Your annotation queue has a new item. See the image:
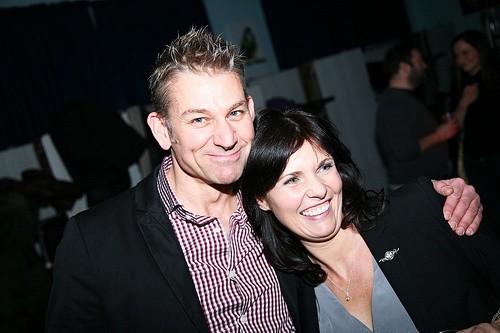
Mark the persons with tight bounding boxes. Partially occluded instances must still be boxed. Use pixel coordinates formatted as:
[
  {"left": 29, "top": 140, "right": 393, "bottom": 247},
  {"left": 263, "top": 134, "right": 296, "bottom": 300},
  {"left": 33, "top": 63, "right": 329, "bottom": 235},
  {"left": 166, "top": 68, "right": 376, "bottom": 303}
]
[{"left": 0, "top": 25, "right": 500, "bottom": 333}]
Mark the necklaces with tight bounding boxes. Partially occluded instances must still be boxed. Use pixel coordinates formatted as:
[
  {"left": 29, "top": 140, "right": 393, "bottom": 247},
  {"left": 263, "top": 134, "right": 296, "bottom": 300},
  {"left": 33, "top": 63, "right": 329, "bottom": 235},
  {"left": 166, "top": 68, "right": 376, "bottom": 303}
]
[{"left": 307, "top": 223, "right": 356, "bottom": 301}]
